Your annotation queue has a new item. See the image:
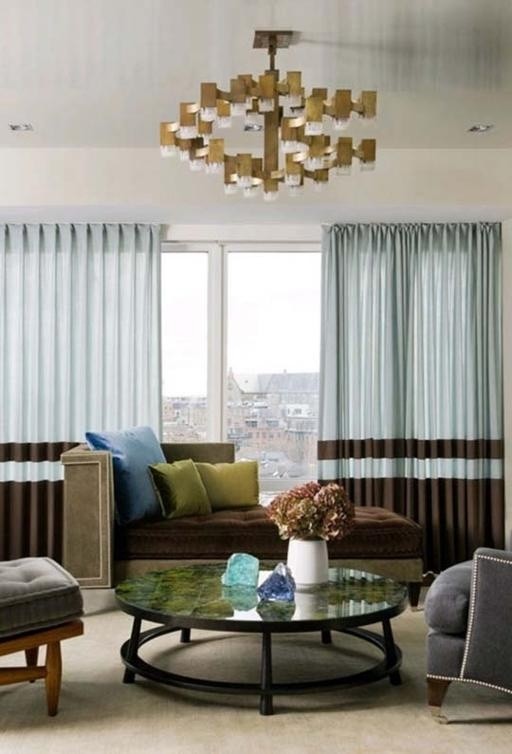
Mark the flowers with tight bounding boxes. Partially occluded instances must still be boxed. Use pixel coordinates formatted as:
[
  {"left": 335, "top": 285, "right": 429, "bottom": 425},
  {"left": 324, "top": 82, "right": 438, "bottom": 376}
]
[{"left": 265, "top": 477, "right": 359, "bottom": 543}]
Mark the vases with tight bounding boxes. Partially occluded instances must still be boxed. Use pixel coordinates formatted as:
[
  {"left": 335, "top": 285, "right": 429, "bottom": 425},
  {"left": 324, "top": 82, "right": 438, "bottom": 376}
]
[{"left": 285, "top": 536, "right": 329, "bottom": 587}]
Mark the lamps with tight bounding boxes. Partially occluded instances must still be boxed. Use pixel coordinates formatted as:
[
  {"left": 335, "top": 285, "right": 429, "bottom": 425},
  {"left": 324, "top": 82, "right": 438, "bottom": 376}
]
[{"left": 155, "top": 31, "right": 380, "bottom": 199}]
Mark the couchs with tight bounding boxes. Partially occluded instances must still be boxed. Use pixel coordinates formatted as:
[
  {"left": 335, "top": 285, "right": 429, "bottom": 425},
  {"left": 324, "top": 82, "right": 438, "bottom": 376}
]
[{"left": 60, "top": 435, "right": 426, "bottom": 616}]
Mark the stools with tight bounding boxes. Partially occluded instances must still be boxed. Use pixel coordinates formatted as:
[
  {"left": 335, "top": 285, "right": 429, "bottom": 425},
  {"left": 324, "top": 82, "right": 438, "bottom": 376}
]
[{"left": 0, "top": 556, "right": 85, "bottom": 716}]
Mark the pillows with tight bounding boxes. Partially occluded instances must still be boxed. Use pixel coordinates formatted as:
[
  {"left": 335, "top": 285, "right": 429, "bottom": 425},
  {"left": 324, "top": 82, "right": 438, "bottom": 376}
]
[{"left": 86, "top": 424, "right": 259, "bottom": 525}]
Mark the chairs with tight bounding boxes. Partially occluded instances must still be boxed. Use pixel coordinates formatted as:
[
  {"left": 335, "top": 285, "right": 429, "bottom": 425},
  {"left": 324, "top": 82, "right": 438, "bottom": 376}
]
[{"left": 423, "top": 540, "right": 511, "bottom": 718}]
